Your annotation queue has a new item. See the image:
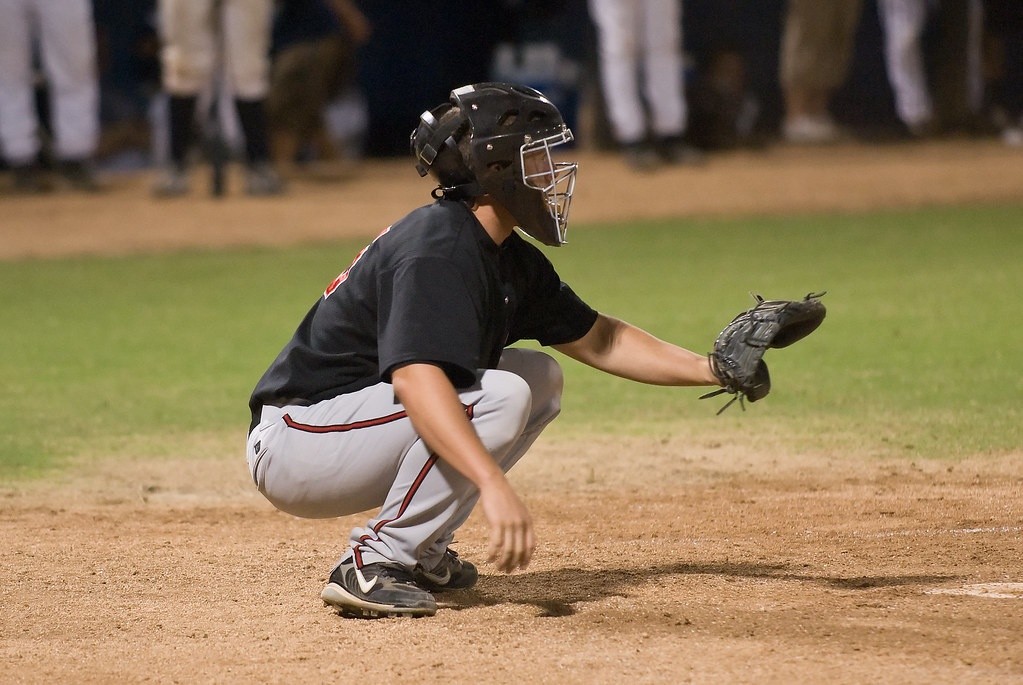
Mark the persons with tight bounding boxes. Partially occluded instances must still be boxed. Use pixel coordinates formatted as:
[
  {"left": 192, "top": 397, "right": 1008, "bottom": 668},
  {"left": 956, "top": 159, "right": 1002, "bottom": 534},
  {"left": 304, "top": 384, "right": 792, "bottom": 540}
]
[
  {"left": 2, "top": 1, "right": 1008, "bottom": 202},
  {"left": 247, "top": 75, "right": 788, "bottom": 622}
]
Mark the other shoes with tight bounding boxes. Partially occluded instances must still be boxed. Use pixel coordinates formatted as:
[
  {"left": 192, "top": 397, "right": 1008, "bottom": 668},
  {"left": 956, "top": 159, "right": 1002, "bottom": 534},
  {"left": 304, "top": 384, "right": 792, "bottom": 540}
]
[
  {"left": 623, "top": 138, "right": 703, "bottom": 175},
  {"left": 12, "top": 159, "right": 108, "bottom": 196},
  {"left": 783, "top": 115, "right": 840, "bottom": 143}
]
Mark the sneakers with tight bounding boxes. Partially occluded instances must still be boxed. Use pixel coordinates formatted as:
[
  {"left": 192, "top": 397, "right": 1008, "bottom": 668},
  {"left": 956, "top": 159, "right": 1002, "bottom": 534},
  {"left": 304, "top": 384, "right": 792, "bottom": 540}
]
[
  {"left": 321, "top": 548, "right": 436, "bottom": 616},
  {"left": 415, "top": 541, "right": 478, "bottom": 591}
]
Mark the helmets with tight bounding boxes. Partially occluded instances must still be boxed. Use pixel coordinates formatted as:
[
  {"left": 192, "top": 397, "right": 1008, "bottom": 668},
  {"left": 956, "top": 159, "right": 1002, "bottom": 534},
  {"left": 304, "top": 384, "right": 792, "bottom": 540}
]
[{"left": 410, "top": 81, "right": 578, "bottom": 247}]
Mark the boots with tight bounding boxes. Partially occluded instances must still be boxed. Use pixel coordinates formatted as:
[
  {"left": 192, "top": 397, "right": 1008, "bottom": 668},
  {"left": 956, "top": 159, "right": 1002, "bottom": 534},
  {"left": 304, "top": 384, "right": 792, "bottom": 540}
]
[
  {"left": 233, "top": 94, "right": 288, "bottom": 195},
  {"left": 157, "top": 93, "right": 196, "bottom": 199}
]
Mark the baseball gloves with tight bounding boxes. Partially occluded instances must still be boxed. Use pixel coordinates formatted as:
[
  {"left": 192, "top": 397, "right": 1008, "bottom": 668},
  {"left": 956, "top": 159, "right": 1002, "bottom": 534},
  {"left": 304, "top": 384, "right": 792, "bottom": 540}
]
[{"left": 698, "top": 288, "right": 833, "bottom": 420}]
